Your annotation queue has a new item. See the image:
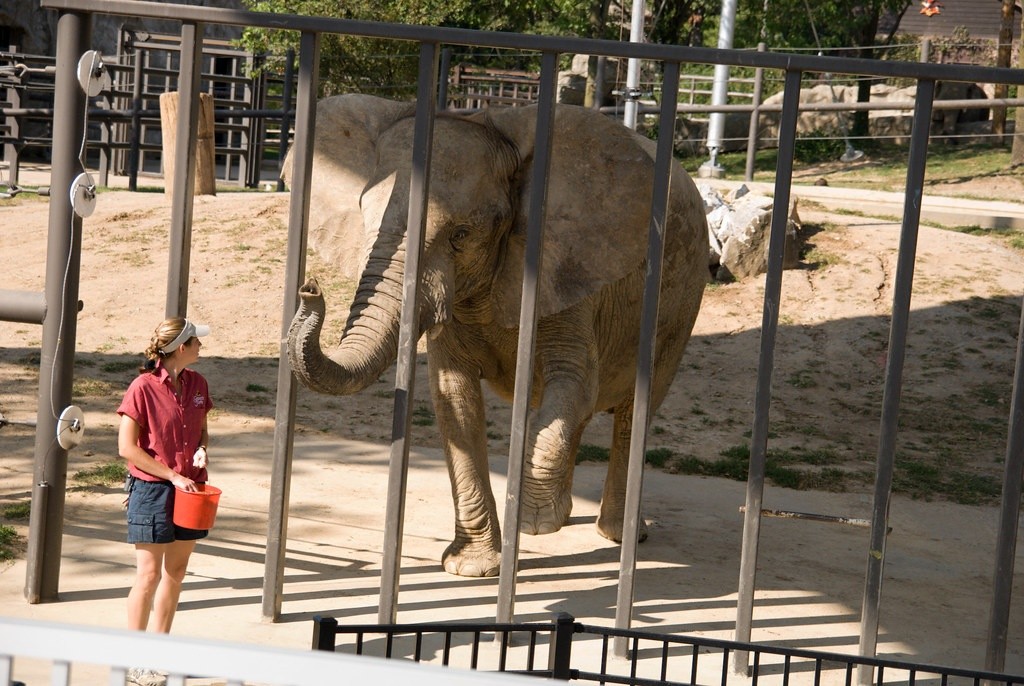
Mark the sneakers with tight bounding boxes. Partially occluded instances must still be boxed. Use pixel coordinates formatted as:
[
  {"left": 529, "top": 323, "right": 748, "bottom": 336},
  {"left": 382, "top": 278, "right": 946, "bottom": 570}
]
[{"left": 126, "top": 664, "right": 170, "bottom": 686}]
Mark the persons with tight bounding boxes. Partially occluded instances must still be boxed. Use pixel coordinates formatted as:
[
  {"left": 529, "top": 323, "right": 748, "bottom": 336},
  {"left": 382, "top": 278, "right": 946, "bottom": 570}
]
[{"left": 116, "top": 317, "right": 216, "bottom": 686}]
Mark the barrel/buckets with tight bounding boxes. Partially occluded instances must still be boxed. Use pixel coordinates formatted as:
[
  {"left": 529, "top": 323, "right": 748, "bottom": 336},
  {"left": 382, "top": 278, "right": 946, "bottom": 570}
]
[{"left": 172, "top": 464, "right": 222, "bottom": 530}]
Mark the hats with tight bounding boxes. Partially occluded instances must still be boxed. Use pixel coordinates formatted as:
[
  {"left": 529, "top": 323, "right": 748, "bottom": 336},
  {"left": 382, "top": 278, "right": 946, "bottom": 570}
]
[{"left": 158, "top": 318, "right": 210, "bottom": 353}]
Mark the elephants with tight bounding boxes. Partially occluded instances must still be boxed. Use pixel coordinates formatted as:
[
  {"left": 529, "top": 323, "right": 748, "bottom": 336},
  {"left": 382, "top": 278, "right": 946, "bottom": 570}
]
[{"left": 279, "top": 90, "right": 711, "bottom": 577}]
